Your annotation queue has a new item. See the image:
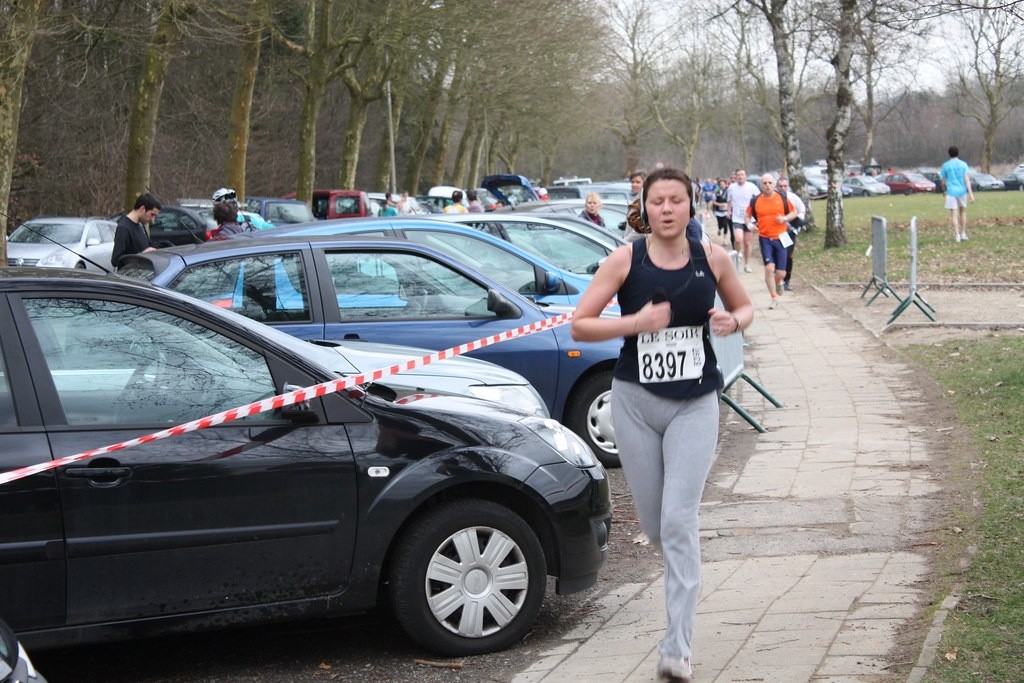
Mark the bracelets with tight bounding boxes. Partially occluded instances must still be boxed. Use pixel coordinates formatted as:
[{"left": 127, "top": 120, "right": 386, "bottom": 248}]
[
  {"left": 633, "top": 312, "right": 639, "bottom": 336},
  {"left": 733, "top": 315, "right": 740, "bottom": 332},
  {"left": 784, "top": 217, "right": 787, "bottom": 222},
  {"left": 747, "top": 223, "right": 754, "bottom": 230}
]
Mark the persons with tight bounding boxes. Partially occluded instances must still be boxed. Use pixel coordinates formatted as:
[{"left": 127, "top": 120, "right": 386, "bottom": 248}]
[
  {"left": 538, "top": 187, "right": 551, "bottom": 201},
  {"left": 941, "top": 146, "right": 975, "bottom": 242},
  {"left": 571, "top": 169, "right": 754, "bottom": 683},
  {"left": 848, "top": 166, "right": 895, "bottom": 177},
  {"left": 775, "top": 178, "right": 807, "bottom": 291},
  {"left": 692, "top": 173, "right": 743, "bottom": 259},
  {"left": 578, "top": 192, "right": 606, "bottom": 229},
  {"left": 111, "top": 193, "right": 162, "bottom": 271},
  {"left": 727, "top": 169, "right": 761, "bottom": 274},
  {"left": 464, "top": 190, "right": 483, "bottom": 213},
  {"left": 496, "top": 200, "right": 506, "bottom": 209},
  {"left": 744, "top": 174, "right": 798, "bottom": 309},
  {"left": 378, "top": 190, "right": 424, "bottom": 217},
  {"left": 624, "top": 170, "right": 651, "bottom": 243},
  {"left": 209, "top": 198, "right": 248, "bottom": 237},
  {"left": 443, "top": 191, "right": 469, "bottom": 214}
]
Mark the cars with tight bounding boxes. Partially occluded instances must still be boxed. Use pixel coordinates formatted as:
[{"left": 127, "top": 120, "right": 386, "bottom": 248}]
[
  {"left": 1, "top": 173, "right": 648, "bottom": 472},
  {"left": 744, "top": 156, "right": 1024, "bottom": 204},
  {"left": 1, "top": 267, "right": 617, "bottom": 667}
]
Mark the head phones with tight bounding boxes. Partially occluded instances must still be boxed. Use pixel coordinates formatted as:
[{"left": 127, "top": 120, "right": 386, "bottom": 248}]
[{"left": 642, "top": 182, "right": 696, "bottom": 224}]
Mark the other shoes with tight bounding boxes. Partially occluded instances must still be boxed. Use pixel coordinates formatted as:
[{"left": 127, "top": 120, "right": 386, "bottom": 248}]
[
  {"left": 776, "top": 280, "right": 784, "bottom": 296},
  {"left": 659, "top": 655, "right": 692, "bottom": 682},
  {"left": 784, "top": 281, "right": 793, "bottom": 291},
  {"left": 744, "top": 265, "right": 752, "bottom": 273},
  {"left": 769, "top": 299, "right": 778, "bottom": 309},
  {"left": 962, "top": 234, "right": 969, "bottom": 241},
  {"left": 955, "top": 236, "right": 962, "bottom": 242}
]
